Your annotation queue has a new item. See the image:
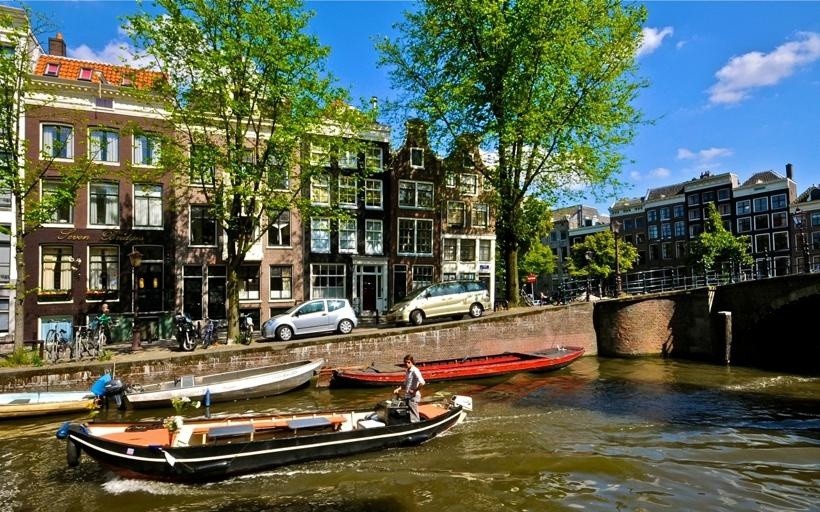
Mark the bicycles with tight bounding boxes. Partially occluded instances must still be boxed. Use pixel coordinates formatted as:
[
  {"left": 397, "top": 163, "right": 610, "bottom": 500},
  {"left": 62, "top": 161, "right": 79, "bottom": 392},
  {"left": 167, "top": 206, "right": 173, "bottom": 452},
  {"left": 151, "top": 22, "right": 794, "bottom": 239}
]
[
  {"left": 46, "top": 317, "right": 110, "bottom": 365},
  {"left": 201, "top": 317, "right": 220, "bottom": 349},
  {"left": 239, "top": 311, "right": 256, "bottom": 346}
]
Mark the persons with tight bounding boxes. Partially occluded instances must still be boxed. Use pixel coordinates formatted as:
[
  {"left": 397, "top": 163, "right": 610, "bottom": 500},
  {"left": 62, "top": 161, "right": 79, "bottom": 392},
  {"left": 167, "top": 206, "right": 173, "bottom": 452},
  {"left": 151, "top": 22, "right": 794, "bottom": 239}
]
[{"left": 394, "top": 354, "right": 425, "bottom": 422}]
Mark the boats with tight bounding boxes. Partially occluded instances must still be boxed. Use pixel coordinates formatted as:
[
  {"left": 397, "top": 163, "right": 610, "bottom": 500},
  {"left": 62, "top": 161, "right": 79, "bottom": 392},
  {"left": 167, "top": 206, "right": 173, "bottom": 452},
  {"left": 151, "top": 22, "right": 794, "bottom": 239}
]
[
  {"left": 0, "top": 390, "right": 104, "bottom": 420},
  {"left": 54, "top": 393, "right": 475, "bottom": 485},
  {"left": 332, "top": 345, "right": 586, "bottom": 389},
  {"left": 103, "top": 356, "right": 330, "bottom": 411}
]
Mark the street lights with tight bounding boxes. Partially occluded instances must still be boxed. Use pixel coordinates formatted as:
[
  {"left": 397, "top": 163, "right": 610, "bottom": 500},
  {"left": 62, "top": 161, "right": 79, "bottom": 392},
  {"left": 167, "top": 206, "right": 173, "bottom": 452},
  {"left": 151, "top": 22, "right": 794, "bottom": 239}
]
[
  {"left": 611, "top": 219, "right": 623, "bottom": 299},
  {"left": 791, "top": 206, "right": 814, "bottom": 273},
  {"left": 127, "top": 246, "right": 144, "bottom": 352}
]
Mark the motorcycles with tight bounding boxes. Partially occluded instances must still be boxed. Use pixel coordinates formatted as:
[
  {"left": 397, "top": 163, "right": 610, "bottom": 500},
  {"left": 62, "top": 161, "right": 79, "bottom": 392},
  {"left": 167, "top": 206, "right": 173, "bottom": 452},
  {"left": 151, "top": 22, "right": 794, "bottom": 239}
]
[{"left": 173, "top": 312, "right": 200, "bottom": 352}]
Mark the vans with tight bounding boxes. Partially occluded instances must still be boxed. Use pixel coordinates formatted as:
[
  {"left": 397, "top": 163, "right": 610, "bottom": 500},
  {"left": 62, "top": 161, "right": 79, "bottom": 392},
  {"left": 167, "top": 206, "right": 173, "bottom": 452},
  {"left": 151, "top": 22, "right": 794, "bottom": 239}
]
[{"left": 384, "top": 279, "right": 491, "bottom": 327}]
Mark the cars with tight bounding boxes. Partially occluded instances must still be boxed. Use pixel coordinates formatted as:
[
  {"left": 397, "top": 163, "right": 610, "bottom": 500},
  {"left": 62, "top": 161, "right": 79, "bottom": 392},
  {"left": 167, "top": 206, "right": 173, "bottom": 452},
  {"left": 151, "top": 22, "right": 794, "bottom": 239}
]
[{"left": 260, "top": 296, "right": 360, "bottom": 342}]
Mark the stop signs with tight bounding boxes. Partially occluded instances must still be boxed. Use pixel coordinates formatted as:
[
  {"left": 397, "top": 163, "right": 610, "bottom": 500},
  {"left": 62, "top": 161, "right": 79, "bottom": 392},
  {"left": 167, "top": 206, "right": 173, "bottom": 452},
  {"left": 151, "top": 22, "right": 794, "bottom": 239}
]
[{"left": 527, "top": 274, "right": 536, "bottom": 283}]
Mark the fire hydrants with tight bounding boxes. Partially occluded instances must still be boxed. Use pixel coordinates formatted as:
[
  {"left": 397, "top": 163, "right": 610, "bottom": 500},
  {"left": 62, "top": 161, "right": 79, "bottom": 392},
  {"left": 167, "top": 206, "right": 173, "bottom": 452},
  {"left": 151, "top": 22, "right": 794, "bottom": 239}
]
[{"left": 130, "top": 320, "right": 145, "bottom": 352}]
